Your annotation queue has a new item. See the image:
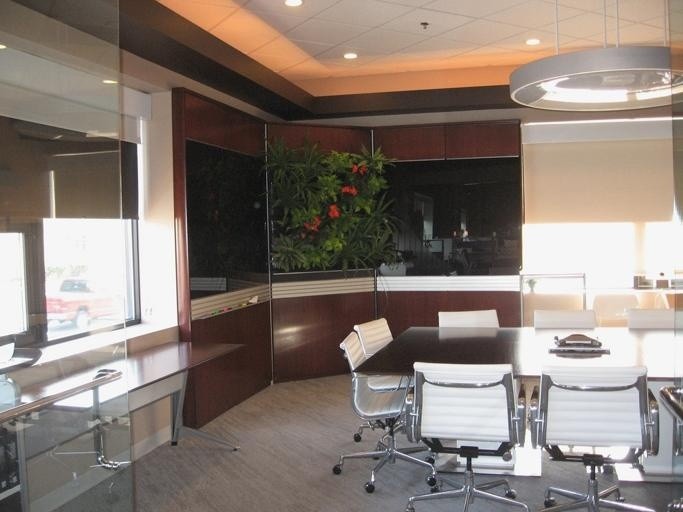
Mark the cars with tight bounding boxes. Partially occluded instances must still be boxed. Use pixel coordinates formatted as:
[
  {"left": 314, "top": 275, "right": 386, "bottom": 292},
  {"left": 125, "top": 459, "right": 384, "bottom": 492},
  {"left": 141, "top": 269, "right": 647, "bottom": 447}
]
[{"left": 45, "top": 276, "right": 116, "bottom": 330}]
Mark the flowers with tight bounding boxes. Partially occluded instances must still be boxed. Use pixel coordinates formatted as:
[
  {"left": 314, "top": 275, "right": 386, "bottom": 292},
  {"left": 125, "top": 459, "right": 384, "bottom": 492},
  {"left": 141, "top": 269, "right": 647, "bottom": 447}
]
[{"left": 256, "top": 134, "right": 400, "bottom": 272}]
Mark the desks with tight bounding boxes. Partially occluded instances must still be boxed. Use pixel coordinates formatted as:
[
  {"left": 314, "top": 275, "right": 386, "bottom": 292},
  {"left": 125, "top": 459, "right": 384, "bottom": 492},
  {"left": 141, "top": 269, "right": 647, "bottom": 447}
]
[
  {"left": 354, "top": 326, "right": 682, "bottom": 484},
  {"left": 19, "top": 340, "right": 245, "bottom": 470}
]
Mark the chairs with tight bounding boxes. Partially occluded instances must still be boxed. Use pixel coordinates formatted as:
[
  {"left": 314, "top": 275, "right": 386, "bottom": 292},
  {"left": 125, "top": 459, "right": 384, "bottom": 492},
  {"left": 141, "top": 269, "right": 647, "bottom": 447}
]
[
  {"left": 535, "top": 309, "right": 597, "bottom": 327},
  {"left": 354, "top": 319, "right": 416, "bottom": 443},
  {"left": 379, "top": 225, "right": 522, "bottom": 275},
  {"left": 660, "top": 386, "right": 682, "bottom": 509},
  {"left": 627, "top": 310, "right": 677, "bottom": 327},
  {"left": 190, "top": 250, "right": 227, "bottom": 292},
  {"left": 405, "top": 361, "right": 530, "bottom": 512},
  {"left": 439, "top": 310, "right": 498, "bottom": 328},
  {"left": 339, "top": 331, "right": 436, "bottom": 492},
  {"left": 537, "top": 366, "right": 657, "bottom": 511}
]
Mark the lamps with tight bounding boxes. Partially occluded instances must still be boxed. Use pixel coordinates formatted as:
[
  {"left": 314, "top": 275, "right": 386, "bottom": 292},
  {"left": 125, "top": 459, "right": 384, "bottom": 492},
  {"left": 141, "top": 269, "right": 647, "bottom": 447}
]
[{"left": 510, "top": 0, "right": 683, "bottom": 112}]
